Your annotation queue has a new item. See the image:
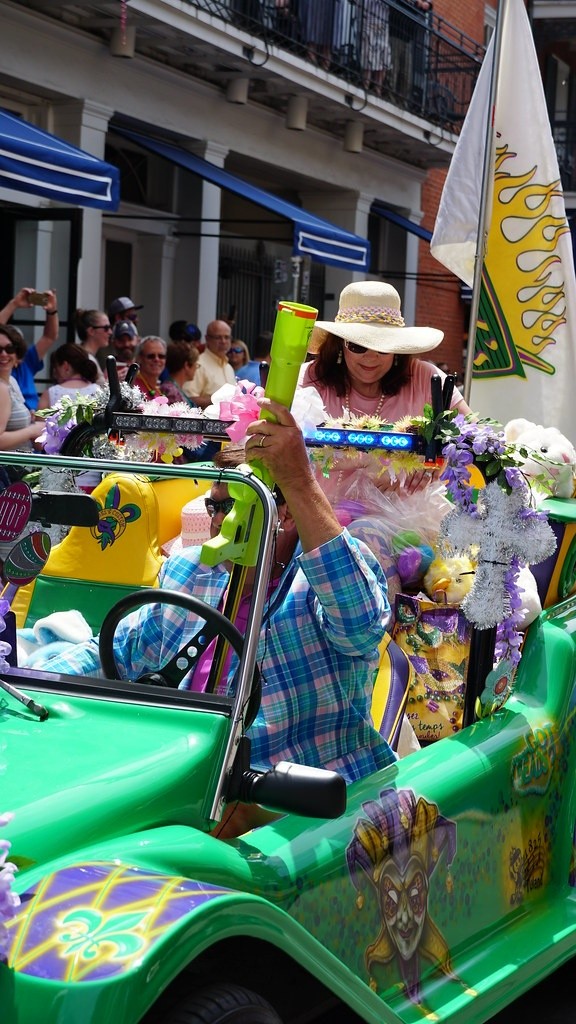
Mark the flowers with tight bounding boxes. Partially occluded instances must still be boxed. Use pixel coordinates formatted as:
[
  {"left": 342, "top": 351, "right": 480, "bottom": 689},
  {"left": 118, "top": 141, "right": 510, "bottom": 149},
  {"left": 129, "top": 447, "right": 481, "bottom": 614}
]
[
  {"left": 36, "top": 409, "right": 70, "bottom": 453},
  {"left": 444, "top": 414, "right": 551, "bottom": 666},
  {"left": 319, "top": 415, "right": 429, "bottom": 475}
]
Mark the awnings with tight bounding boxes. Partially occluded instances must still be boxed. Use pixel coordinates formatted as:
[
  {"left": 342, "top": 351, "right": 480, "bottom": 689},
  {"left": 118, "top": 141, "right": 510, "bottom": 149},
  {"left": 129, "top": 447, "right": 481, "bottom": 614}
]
[
  {"left": 370, "top": 205, "right": 465, "bottom": 284},
  {"left": 0, "top": 108, "right": 120, "bottom": 213},
  {"left": 108, "top": 128, "right": 371, "bottom": 274}
]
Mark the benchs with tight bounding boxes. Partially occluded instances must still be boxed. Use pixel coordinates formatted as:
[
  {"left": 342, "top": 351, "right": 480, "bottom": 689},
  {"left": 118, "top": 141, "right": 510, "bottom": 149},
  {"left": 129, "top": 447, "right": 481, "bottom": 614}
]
[{"left": 21, "top": 577, "right": 405, "bottom": 764}]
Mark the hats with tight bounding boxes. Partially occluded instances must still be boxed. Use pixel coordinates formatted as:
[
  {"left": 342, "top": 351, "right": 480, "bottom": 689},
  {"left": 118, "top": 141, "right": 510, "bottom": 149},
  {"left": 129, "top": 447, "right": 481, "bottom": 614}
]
[
  {"left": 108, "top": 297, "right": 144, "bottom": 316},
  {"left": 161, "top": 494, "right": 213, "bottom": 557},
  {"left": 307, "top": 281, "right": 445, "bottom": 355},
  {"left": 112, "top": 320, "right": 138, "bottom": 339}
]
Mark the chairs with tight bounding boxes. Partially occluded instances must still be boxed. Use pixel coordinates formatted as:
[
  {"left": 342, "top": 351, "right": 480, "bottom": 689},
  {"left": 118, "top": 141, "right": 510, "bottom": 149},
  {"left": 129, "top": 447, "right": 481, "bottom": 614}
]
[{"left": 280, "top": 14, "right": 449, "bottom": 125}]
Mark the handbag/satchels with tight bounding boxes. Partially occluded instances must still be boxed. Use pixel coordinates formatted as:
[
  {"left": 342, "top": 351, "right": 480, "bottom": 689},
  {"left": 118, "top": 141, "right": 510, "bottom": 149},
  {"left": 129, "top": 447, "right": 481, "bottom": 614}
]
[{"left": 392, "top": 592, "right": 497, "bottom": 743}]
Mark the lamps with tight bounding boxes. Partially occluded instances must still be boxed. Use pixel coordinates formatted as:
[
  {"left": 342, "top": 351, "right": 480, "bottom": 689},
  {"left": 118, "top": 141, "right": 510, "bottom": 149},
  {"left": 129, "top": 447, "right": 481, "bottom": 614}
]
[
  {"left": 283, "top": 92, "right": 307, "bottom": 134},
  {"left": 226, "top": 78, "right": 249, "bottom": 104},
  {"left": 108, "top": 26, "right": 135, "bottom": 58},
  {"left": 344, "top": 121, "right": 367, "bottom": 151}
]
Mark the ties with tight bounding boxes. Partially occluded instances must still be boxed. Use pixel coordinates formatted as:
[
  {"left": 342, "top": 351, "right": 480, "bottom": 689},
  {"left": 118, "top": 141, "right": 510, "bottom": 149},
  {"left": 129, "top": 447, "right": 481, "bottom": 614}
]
[{"left": 186, "top": 577, "right": 281, "bottom": 695}]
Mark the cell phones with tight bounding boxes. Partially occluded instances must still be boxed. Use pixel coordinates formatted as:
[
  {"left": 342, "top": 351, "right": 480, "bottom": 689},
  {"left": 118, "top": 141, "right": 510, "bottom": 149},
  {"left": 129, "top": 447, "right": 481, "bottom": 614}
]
[
  {"left": 227, "top": 305, "right": 236, "bottom": 320},
  {"left": 27, "top": 291, "right": 49, "bottom": 306}
]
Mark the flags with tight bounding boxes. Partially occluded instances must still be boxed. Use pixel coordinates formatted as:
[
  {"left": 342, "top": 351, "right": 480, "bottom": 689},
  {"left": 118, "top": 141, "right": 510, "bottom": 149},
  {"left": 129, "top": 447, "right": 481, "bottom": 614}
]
[{"left": 429, "top": 0, "right": 576, "bottom": 450}]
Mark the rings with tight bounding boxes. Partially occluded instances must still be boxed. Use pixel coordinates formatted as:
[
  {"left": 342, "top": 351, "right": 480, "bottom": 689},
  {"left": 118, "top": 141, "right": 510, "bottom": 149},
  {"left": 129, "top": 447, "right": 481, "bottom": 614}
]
[
  {"left": 425, "top": 471, "right": 432, "bottom": 478},
  {"left": 260, "top": 435, "right": 266, "bottom": 446}
]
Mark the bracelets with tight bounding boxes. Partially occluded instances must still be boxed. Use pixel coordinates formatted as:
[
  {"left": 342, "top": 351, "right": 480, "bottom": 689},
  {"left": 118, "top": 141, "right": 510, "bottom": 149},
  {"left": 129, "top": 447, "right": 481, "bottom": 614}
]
[{"left": 46, "top": 311, "right": 58, "bottom": 314}]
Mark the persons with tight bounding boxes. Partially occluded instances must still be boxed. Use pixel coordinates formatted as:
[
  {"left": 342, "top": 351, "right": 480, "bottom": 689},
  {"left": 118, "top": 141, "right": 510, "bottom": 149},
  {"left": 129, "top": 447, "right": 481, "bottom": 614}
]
[
  {"left": 34, "top": 398, "right": 392, "bottom": 840},
  {"left": 38, "top": 343, "right": 104, "bottom": 410},
  {"left": 357, "top": 0, "right": 429, "bottom": 95},
  {"left": 0, "top": 324, "right": 46, "bottom": 452},
  {"left": 0, "top": 288, "right": 59, "bottom": 411},
  {"left": 104, "top": 297, "right": 202, "bottom": 408},
  {"left": 299, "top": 0, "right": 336, "bottom": 72},
  {"left": 185, "top": 320, "right": 237, "bottom": 405},
  {"left": 228, "top": 339, "right": 260, "bottom": 386},
  {"left": 291, "top": 280, "right": 479, "bottom": 606},
  {"left": 73, "top": 309, "right": 112, "bottom": 383}
]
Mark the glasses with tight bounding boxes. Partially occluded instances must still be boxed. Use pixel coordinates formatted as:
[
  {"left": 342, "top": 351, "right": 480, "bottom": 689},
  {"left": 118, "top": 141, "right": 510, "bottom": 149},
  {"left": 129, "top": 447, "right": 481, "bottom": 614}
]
[
  {"left": 208, "top": 335, "right": 232, "bottom": 341},
  {"left": 228, "top": 348, "right": 244, "bottom": 353},
  {"left": 0, "top": 344, "right": 16, "bottom": 354},
  {"left": 192, "top": 362, "right": 201, "bottom": 367},
  {"left": 120, "top": 314, "right": 136, "bottom": 320},
  {"left": 92, "top": 325, "right": 111, "bottom": 330},
  {"left": 142, "top": 353, "right": 166, "bottom": 359},
  {"left": 344, "top": 340, "right": 388, "bottom": 354},
  {"left": 205, "top": 498, "right": 236, "bottom": 517}
]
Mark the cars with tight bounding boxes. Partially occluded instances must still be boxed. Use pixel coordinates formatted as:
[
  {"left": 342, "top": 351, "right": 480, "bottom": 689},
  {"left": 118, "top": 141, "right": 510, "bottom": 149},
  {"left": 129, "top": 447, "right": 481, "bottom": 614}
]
[{"left": 1, "top": 453, "right": 576, "bottom": 1024}]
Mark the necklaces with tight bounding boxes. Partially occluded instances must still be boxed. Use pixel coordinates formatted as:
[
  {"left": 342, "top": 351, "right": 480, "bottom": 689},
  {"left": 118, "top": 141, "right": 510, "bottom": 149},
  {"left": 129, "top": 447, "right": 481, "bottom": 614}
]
[
  {"left": 335, "top": 392, "right": 385, "bottom": 501},
  {"left": 138, "top": 374, "right": 157, "bottom": 395}
]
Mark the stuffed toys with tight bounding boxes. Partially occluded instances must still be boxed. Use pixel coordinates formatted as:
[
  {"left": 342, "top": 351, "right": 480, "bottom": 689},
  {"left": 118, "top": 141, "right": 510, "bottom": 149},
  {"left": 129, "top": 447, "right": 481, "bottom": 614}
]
[
  {"left": 426, "top": 537, "right": 542, "bottom": 628},
  {"left": 505, "top": 418, "right": 576, "bottom": 499}
]
